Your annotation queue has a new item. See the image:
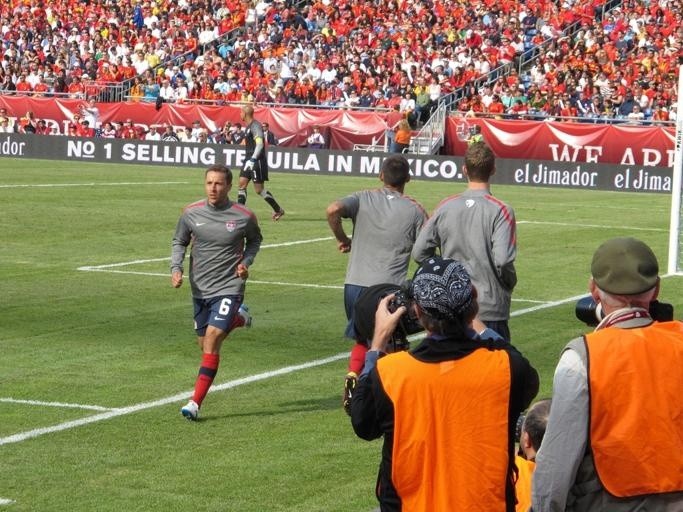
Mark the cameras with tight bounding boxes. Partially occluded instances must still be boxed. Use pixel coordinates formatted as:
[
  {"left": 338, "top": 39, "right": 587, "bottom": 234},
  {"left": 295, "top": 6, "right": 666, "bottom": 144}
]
[
  {"left": 378, "top": 281, "right": 424, "bottom": 336},
  {"left": 574, "top": 295, "right": 675, "bottom": 325}
]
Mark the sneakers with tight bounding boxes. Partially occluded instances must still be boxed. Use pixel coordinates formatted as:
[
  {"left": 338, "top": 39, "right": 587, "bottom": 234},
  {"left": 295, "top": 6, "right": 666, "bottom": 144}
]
[
  {"left": 238, "top": 304, "right": 251, "bottom": 327},
  {"left": 182, "top": 400, "right": 199, "bottom": 420},
  {"left": 272, "top": 208, "right": 284, "bottom": 220},
  {"left": 344, "top": 371, "right": 357, "bottom": 415}
]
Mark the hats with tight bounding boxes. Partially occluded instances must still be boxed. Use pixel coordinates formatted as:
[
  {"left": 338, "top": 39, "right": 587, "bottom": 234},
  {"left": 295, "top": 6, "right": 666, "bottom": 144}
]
[
  {"left": 411, "top": 256, "right": 472, "bottom": 319},
  {"left": 591, "top": 238, "right": 658, "bottom": 295}
]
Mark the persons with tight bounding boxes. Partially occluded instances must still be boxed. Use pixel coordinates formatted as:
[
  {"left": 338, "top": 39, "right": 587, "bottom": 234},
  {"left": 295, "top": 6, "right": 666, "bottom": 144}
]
[
  {"left": 171, "top": 166, "right": 263, "bottom": 420},
  {"left": 234, "top": 107, "right": 286, "bottom": 220},
  {"left": 326, "top": 155, "right": 429, "bottom": 415},
  {"left": 531, "top": 237, "right": 682, "bottom": 510},
  {"left": 411, "top": 142, "right": 517, "bottom": 345},
  {"left": 350, "top": 258, "right": 539, "bottom": 509},
  {"left": 0, "top": 0, "right": 682, "bottom": 153},
  {"left": 512, "top": 401, "right": 553, "bottom": 509}
]
